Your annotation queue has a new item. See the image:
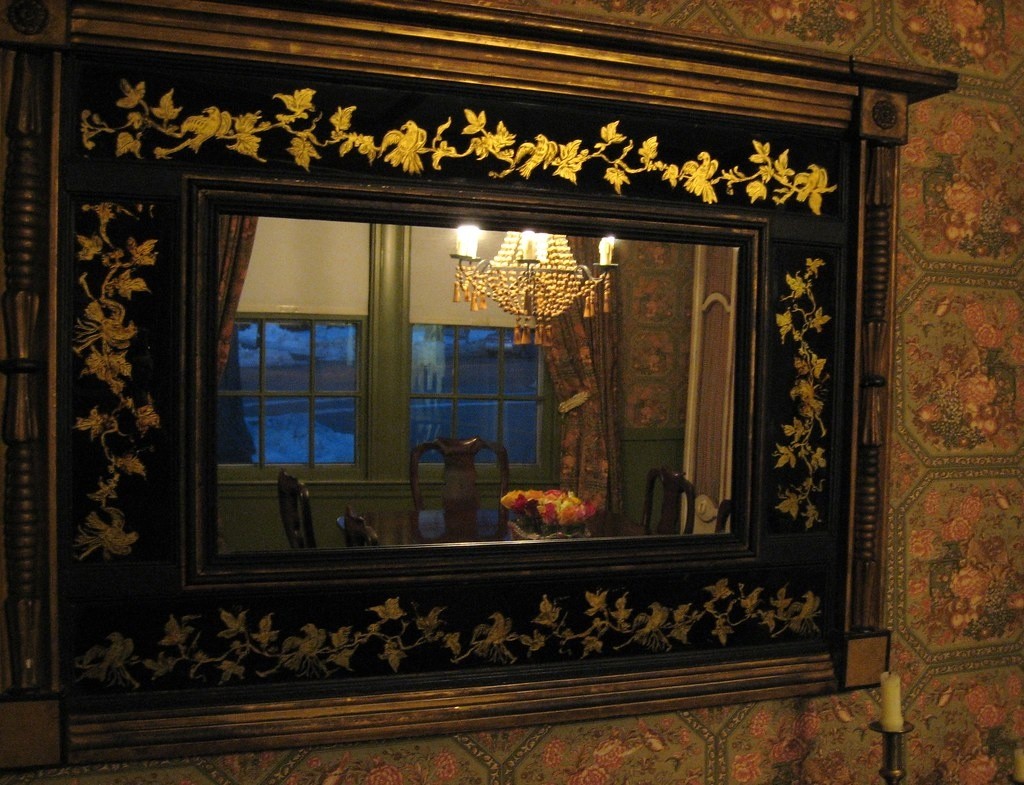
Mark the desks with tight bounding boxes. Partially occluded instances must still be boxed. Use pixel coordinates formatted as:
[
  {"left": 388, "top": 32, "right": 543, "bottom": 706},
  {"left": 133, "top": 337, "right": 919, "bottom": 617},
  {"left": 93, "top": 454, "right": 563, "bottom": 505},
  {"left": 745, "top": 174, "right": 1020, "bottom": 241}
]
[{"left": 334, "top": 513, "right": 645, "bottom": 542}]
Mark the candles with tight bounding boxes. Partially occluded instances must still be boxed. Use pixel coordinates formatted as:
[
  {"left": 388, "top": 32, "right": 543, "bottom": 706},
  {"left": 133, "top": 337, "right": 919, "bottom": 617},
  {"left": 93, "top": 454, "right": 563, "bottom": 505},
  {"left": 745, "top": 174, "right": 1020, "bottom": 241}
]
[{"left": 881, "top": 670, "right": 905, "bottom": 728}]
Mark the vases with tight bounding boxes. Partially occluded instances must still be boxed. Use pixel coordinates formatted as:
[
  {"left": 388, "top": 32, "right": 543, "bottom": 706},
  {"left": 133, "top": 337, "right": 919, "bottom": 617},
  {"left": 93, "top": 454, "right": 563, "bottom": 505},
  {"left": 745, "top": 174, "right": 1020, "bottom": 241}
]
[{"left": 506, "top": 519, "right": 595, "bottom": 541}]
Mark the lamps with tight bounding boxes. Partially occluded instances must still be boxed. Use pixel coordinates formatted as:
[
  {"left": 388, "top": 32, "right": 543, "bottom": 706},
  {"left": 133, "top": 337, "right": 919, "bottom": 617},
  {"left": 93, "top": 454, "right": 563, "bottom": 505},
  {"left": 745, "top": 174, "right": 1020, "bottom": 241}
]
[{"left": 448, "top": 223, "right": 617, "bottom": 346}]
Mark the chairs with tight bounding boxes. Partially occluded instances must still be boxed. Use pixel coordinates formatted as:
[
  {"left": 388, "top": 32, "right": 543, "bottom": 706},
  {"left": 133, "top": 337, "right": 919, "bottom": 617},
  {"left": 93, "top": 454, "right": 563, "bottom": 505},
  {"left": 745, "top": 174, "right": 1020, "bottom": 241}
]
[
  {"left": 641, "top": 469, "right": 700, "bottom": 536},
  {"left": 409, "top": 437, "right": 510, "bottom": 512},
  {"left": 279, "top": 470, "right": 316, "bottom": 547},
  {"left": 345, "top": 504, "right": 381, "bottom": 546}
]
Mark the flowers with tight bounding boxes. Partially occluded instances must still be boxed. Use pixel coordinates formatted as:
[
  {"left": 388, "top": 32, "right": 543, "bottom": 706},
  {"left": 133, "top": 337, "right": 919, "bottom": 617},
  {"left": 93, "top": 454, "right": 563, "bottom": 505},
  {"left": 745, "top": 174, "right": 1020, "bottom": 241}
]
[{"left": 500, "top": 489, "right": 606, "bottom": 530}]
